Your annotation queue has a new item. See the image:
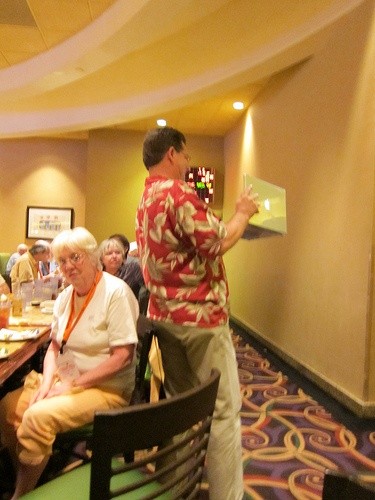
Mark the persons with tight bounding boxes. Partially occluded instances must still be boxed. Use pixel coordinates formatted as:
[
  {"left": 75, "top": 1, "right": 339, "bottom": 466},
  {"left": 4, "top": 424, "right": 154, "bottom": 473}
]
[
  {"left": 0, "top": 233, "right": 145, "bottom": 302},
  {"left": 0, "top": 227, "right": 140, "bottom": 500},
  {"left": 135, "top": 128, "right": 261, "bottom": 500}
]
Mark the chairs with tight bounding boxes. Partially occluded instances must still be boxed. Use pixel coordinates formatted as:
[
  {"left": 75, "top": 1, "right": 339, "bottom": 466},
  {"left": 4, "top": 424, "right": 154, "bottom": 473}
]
[
  {"left": 35, "top": 315, "right": 155, "bottom": 489},
  {"left": 16, "top": 368, "right": 221, "bottom": 500}
]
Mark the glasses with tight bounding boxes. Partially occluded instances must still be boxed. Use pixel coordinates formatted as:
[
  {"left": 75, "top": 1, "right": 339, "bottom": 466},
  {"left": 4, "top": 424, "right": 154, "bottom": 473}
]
[{"left": 55, "top": 253, "right": 87, "bottom": 267}]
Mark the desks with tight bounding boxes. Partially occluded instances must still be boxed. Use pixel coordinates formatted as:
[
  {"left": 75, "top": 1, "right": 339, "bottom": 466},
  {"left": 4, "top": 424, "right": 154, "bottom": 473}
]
[{"left": 0, "top": 324, "right": 52, "bottom": 483}]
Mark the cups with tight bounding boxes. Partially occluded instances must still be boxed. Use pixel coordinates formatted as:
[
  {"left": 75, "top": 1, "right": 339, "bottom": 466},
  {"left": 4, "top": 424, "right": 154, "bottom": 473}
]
[
  {"left": 0, "top": 303, "right": 12, "bottom": 330},
  {"left": 12, "top": 297, "right": 23, "bottom": 317},
  {"left": 21, "top": 277, "right": 59, "bottom": 312}
]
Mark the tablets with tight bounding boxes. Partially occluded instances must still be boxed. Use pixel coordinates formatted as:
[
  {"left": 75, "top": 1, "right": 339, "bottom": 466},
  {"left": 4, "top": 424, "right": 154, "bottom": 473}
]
[{"left": 243, "top": 174, "right": 287, "bottom": 235}]
